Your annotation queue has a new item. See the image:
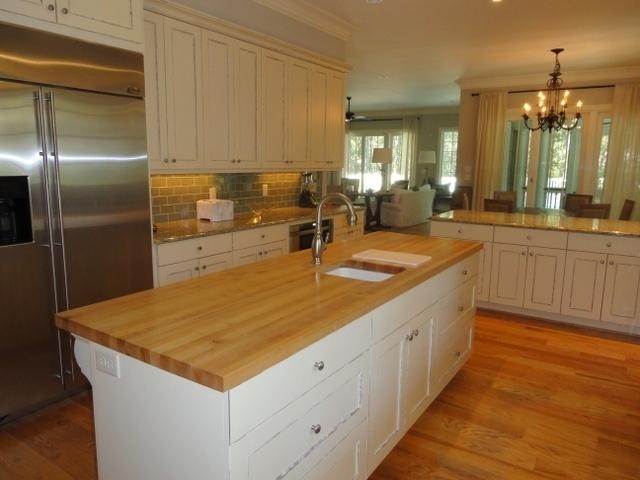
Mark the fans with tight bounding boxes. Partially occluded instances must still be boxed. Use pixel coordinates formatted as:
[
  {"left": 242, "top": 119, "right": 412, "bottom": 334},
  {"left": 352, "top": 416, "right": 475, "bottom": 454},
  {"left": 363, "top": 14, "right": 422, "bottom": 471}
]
[{"left": 343, "top": 93, "right": 370, "bottom": 123}]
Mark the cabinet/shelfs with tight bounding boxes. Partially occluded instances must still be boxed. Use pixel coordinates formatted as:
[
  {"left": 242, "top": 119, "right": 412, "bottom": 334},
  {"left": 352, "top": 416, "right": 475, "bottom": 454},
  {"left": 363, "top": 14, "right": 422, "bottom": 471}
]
[
  {"left": 366, "top": 277, "right": 432, "bottom": 478},
  {"left": 202, "top": 12, "right": 267, "bottom": 181},
  {"left": 230, "top": 224, "right": 294, "bottom": 268},
  {"left": 312, "top": 49, "right": 350, "bottom": 178},
  {"left": 331, "top": 212, "right": 365, "bottom": 242},
  {"left": 493, "top": 223, "right": 563, "bottom": 324},
  {"left": 75, "top": 317, "right": 366, "bottom": 479},
  {"left": 143, "top": 0, "right": 204, "bottom": 175},
  {"left": 148, "top": 228, "right": 231, "bottom": 287},
  {"left": 564, "top": 235, "right": 639, "bottom": 342},
  {"left": 427, "top": 253, "right": 483, "bottom": 405},
  {"left": 1, "top": 2, "right": 145, "bottom": 52},
  {"left": 265, "top": 33, "right": 313, "bottom": 178},
  {"left": 431, "top": 221, "right": 492, "bottom": 311}
]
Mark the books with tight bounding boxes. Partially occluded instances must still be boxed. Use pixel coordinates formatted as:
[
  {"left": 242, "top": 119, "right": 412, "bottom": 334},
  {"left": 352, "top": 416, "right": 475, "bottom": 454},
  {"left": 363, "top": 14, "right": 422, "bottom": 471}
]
[{"left": 351, "top": 248, "right": 432, "bottom": 266}]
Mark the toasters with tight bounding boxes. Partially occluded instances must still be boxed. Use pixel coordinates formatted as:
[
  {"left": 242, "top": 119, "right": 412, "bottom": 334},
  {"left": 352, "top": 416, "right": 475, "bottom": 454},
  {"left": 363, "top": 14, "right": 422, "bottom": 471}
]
[{"left": 196, "top": 199, "right": 234, "bottom": 222}]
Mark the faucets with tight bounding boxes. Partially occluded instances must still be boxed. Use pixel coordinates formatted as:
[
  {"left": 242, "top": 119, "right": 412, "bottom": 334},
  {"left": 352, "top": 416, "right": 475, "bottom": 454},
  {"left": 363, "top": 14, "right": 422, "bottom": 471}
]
[{"left": 311, "top": 192, "right": 357, "bottom": 266}]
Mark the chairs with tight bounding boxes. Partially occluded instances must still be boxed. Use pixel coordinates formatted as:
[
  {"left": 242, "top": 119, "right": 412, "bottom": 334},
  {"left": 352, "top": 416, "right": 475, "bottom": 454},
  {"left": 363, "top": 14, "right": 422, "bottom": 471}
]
[
  {"left": 482, "top": 188, "right": 636, "bottom": 222},
  {"left": 327, "top": 177, "right": 474, "bottom": 231}
]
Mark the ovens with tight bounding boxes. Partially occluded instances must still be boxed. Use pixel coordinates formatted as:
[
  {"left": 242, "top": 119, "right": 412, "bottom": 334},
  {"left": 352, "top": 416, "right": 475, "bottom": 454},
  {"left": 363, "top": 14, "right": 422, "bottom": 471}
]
[{"left": 289, "top": 218, "right": 334, "bottom": 253}]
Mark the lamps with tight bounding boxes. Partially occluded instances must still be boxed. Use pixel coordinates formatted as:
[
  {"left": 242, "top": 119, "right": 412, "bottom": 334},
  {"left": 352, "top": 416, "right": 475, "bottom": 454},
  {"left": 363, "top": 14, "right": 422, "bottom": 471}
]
[
  {"left": 371, "top": 147, "right": 393, "bottom": 190},
  {"left": 416, "top": 150, "right": 438, "bottom": 186},
  {"left": 520, "top": 47, "right": 586, "bottom": 135}
]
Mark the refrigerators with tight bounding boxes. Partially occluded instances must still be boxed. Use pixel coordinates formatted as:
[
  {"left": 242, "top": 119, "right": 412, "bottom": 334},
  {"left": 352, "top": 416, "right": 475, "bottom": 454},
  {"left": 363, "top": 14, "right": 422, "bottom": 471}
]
[{"left": 0, "top": 19, "right": 156, "bottom": 430}]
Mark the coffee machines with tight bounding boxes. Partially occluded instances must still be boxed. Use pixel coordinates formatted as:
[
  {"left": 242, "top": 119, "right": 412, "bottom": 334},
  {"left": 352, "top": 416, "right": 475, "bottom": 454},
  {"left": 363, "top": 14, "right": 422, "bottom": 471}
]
[{"left": 298, "top": 171, "right": 321, "bottom": 209}]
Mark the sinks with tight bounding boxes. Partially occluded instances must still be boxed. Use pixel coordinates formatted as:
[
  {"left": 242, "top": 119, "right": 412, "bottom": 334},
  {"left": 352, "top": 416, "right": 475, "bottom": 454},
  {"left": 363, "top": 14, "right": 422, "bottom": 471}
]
[{"left": 325, "top": 267, "right": 393, "bottom": 282}]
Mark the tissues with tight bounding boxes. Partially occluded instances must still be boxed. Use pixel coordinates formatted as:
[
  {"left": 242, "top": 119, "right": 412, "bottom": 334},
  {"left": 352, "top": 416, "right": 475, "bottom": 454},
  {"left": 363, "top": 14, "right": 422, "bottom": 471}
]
[{"left": 196, "top": 188, "right": 234, "bottom": 222}]
